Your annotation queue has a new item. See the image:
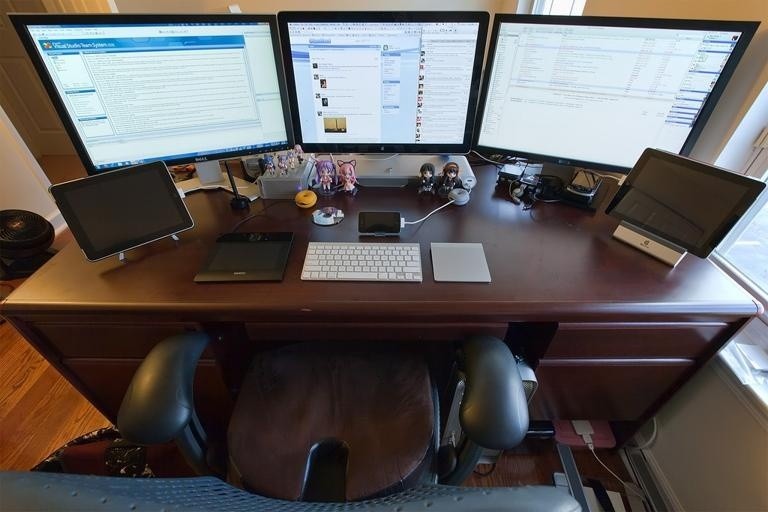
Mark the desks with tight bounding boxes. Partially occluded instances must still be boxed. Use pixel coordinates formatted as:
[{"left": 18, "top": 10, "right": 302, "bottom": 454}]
[{"left": 0, "top": 159, "right": 764, "bottom": 452}]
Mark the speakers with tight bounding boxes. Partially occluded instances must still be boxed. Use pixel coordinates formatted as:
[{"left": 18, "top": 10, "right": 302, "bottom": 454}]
[{"left": 439, "top": 354, "right": 539, "bottom": 479}]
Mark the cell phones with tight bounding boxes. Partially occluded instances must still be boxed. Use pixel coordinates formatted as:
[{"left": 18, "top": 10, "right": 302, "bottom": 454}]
[
  {"left": 567, "top": 171, "right": 602, "bottom": 197},
  {"left": 357, "top": 211, "right": 401, "bottom": 235}
]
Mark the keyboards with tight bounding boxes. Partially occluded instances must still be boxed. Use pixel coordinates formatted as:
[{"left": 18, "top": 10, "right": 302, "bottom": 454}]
[{"left": 300, "top": 242, "right": 423, "bottom": 283}]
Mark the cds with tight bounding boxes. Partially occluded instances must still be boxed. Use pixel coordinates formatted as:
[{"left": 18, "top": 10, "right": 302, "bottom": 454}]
[{"left": 311, "top": 206, "right": 344, "bottom": 226}]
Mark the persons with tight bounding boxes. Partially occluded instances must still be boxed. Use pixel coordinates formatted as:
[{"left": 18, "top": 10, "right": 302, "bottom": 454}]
[
  {"left": 416, "top": 163, "right": 436, "bottom": 195},
  {"left": 277, "top": 155, "right": 288, "bottom": 176},
  {"left": 294, "top": 145, "right": 304, "bottom": 164},
  {"left": 313, "top": 160, "right": 336, "bottom": 193},
  {"left": 287, "top": 149, "right": 296, "bottom": 169},
  {"left": 442, "top": 162, "right": 460, "bottom": 192},
  {"left": 337, "top": 159, "right": 360, "bottom": 195},
  {"left": 263, "top": 156, "right": 275, "bottom": 175}
]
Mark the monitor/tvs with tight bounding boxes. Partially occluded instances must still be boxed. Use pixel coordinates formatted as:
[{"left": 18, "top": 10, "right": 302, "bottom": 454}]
[
  {"left": 471, "top": 13, "right": 761, "bottom": 214},
  {"left": 604, "top": 148, "right": 767, "bottom": 268},
  {"left": 7, "top": 12, "right": 295, "bottom": 202},
  {"left": 276, "top": 10, "right": 489, "bottom": 188},
  {"left": 48, "top": 160, "right": 195, "bottom": 263}
]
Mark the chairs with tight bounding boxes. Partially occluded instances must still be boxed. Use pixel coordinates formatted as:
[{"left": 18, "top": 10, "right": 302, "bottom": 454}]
[{"left": 0, "top": 331, "right": 582, "bottom": 512}]
